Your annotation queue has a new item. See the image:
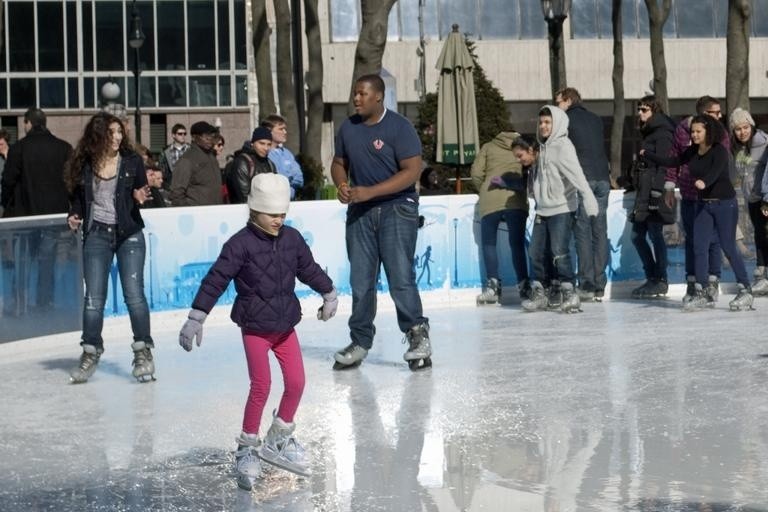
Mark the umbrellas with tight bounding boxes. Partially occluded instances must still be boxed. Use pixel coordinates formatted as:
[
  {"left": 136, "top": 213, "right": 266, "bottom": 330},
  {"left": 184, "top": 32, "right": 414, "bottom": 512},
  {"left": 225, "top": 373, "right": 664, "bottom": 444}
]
[{"left": 435, "top": 23, "right": 478, "bottom": 192}]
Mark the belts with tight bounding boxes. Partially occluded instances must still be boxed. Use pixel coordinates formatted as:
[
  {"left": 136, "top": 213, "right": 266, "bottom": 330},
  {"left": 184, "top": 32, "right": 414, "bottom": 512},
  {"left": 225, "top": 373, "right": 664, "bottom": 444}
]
[{"left": 92, "top": 219, "right": 118, "bottom": 233}]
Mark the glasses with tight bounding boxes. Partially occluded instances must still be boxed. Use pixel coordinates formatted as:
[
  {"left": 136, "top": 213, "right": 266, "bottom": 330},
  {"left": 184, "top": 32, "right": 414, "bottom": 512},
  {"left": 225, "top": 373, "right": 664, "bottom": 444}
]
[
  {"left": 637, "top": 108, "right": 650, "bottom": 113},
  {"left": 556, "top": 101, "right": 564, "bottom": 106},
  {"left": 706, "top": 110, "right": 721, "bottom": 117},
  {"left": 217, "top": 143, "right": 224, "bottom": 147},
  {"left": 177, "top": 133, "right": 186, "bottom": 136}
]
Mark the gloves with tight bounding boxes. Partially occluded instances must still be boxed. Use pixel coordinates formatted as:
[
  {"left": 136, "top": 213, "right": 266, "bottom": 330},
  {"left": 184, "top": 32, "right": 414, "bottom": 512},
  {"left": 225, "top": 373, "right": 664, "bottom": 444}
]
[
  {"left": 490, "top": 175, "right": 508, "bottom": 188},
  {"left": 178, "top": 309, "right": 208, "bottom": 352},
  {"left": 317, "top": 284, "right": 339, "bottom": 322},
  {"left": 647, "top": 188, "right": 663, "bottom": 214}
]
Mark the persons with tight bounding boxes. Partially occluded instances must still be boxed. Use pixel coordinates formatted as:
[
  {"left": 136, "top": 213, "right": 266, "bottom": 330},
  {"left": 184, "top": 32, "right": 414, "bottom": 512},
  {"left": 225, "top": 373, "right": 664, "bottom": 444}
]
[
  {"left": 1, "top": 130, "right": 12, "bottom": 176},
  {"left": 179, "top": 173, "right": 340, "bottom": 493},
  {"left": 64, "top": 111, "right": 160, "bottom": 385},
  {"left": 6, "top": 108, "right": 74, "bottom": 213},
  {"left": 333, "top": 72, "right": 436, "bottom": 373},
  {"left": 463, "top": 87, "right": 768, "bottom": 313},
  {"left": 420, "top": 164, "right": 445, "bottom": 197},
  {"left": 116, "top": 115, "right": 304, "bottom": 205}
]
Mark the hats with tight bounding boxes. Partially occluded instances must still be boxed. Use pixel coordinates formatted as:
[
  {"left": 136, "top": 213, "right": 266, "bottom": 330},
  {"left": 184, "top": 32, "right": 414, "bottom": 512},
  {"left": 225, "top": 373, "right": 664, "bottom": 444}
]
[
  {"left": 250, "top": 126, "right": 272, "bottom": 144},
  {"left": 248, "top": 172, "right": 291, "bottom": 214},
  {"left": 728, "top": 107, "right": 756, "bottom": 136}
]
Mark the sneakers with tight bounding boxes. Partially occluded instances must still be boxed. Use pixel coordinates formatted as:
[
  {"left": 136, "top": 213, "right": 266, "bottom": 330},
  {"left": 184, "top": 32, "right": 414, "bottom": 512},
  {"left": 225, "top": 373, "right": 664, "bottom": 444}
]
[
  {"left": 131, "top": 341, "right": 155, "bottom": 378},
  {"left": 333, "top": 342, "right": 368, "bottom": 365},
  {"left": 234, "top": 430, "right": 261, "bottom": 478},
  {"left": 402, "top": 321, "right": 433, "bottom": 361},
  {"left": 261, "top": 416, "right": 310, "bottom": 471},
  {"left": 68, "top": 343, "right": 104, "bottom": 383},
  {"left": 476, "top": 265, "right": 768, "bottom": 314}
]
